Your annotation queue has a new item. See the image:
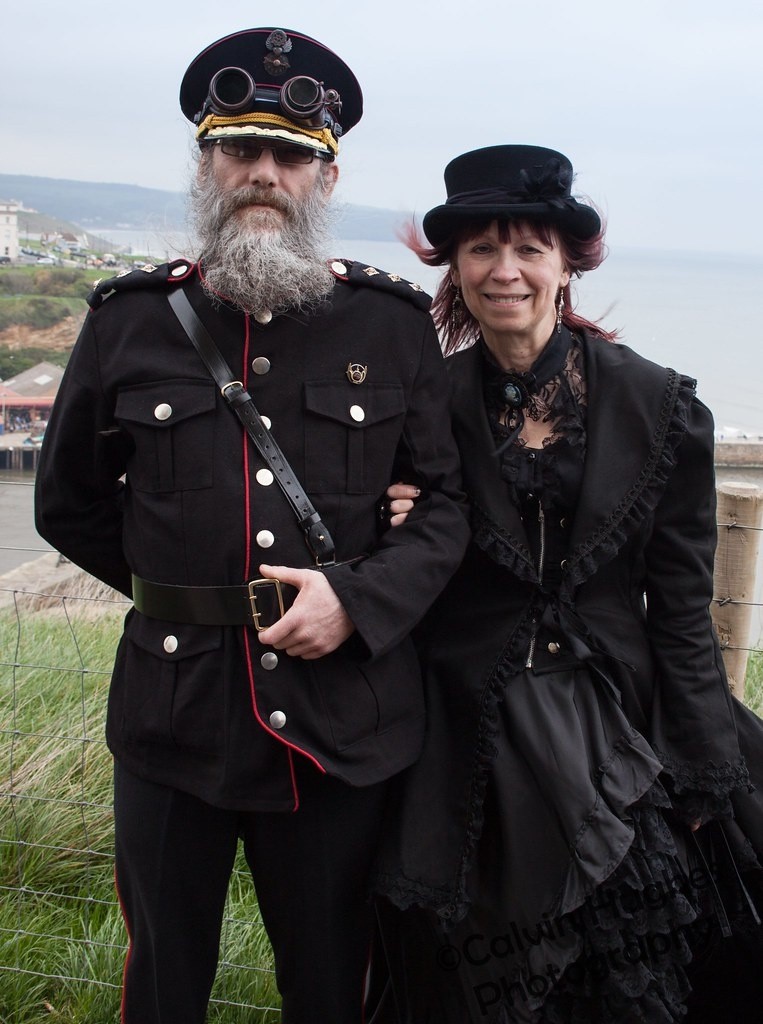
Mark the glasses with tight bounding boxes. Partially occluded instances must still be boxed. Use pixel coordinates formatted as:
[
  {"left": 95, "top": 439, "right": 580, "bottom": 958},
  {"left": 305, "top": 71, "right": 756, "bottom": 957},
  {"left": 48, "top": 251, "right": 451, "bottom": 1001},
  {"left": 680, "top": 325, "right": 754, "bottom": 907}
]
[{"left": 201, "top": 138, "right": 328, "bottom": 166}]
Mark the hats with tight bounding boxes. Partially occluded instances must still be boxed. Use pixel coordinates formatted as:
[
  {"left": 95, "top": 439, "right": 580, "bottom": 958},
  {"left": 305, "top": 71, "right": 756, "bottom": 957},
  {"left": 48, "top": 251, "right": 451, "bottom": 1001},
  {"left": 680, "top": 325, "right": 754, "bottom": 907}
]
[
  {"left": 423, "top": 145, "right": 601, "bottom": 248},
  {"left": 180, "top": 27, "right": 363, "bottom": 153}
]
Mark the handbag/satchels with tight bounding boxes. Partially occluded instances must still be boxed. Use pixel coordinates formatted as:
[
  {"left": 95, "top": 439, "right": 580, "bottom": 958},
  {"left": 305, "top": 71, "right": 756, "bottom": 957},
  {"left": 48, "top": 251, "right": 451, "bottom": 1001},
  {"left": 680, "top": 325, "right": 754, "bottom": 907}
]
[{"left": 691, "top": 817, "right": 763, "bottom": 1024}]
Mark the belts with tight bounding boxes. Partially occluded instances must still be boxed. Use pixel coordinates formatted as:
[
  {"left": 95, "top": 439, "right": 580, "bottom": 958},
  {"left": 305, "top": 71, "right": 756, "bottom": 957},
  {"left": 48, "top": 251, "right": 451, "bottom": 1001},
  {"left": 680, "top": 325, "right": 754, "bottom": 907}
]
[{"left": 132, "top": 555, "right": 388, "bottom": 626}]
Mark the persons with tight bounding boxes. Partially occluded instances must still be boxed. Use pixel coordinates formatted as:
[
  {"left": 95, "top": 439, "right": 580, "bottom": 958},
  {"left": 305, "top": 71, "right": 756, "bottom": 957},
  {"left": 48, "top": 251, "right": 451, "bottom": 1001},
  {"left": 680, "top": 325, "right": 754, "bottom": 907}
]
[
  {"left": 34, "top": 26, "right": 473, "bottom": 1024},
  {"left": 369, "top": 145, "right": 763, "bottom": 1024}
]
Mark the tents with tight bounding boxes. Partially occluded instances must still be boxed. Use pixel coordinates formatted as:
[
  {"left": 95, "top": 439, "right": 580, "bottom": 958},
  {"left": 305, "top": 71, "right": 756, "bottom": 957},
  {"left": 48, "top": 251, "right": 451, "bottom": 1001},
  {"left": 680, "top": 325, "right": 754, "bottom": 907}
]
[{"left": 0, "top": 361, "right": 65, "bottom": 434}]
